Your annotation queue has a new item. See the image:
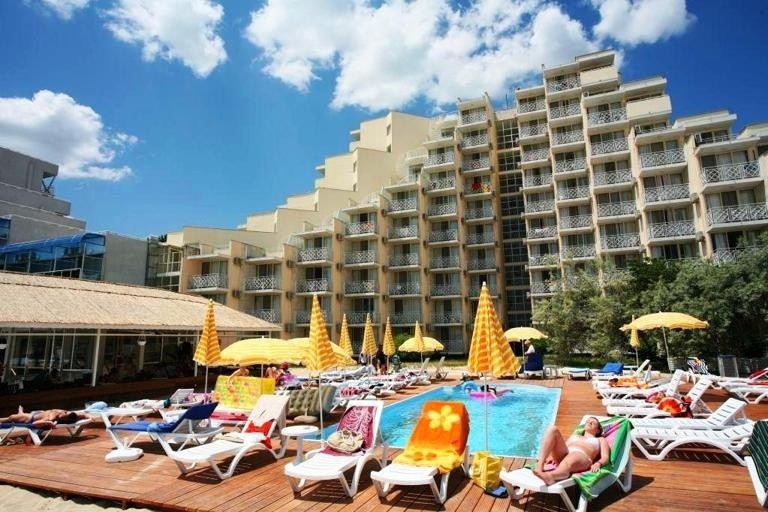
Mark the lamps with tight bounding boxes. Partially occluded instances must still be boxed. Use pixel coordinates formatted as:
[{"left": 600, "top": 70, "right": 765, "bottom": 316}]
[
  {"left": 0, "top": 327, "right": 8, "bottom": 350},
  {"left": 137, "top": 330, "right": 147, "bottom": 347}
]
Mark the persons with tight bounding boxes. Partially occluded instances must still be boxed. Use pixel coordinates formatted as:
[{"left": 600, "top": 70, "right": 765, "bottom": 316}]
[
  {"left": 532, "top": 416, "right": 610, "bottom": 486},
  {"left": 227, "top": 361, "right": 293, "bottom": 392},
  {"left": 480, "top": 385, "right": 512, "bottom": 394},
  {"left": 525, "top": 340, "right": 535, "bottom": 355},
  {"left": 358, "top": 349, "right": 367, "bottom": 366},
  {"left": 0, "top": 405, "right": 85, "bottom": 429},
  {"left": 693, "top": 358, "right": 708, "bottom": 375},
  {"left": 376, "top": 346, "right": 385, "bottom": 371},
  {"left": 391, "top": 351, "right": 401, "bottom": 371}
]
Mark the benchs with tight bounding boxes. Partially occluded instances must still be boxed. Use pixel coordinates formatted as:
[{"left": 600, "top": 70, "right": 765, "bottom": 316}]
[{"left": 297, "top": 356, "right": 448, "bottom": 399}]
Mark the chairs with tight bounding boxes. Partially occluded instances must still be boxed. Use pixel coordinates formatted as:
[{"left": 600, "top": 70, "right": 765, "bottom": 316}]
[
  {"left": 594, "top": 358, "right": 712, "bottom": 418},
  {"left": 109, "top": 401, "right": 247, "bottom": 460},
  {"left": 567, "top": 368, "right": 592, "bottom": 379},
  {"left": 701, "top": 368, "right": 768, "bottom": 404},
  {"left": 627, "top": 398, "right": 768, "bottom": 466},
  {"left": 169, "top": 395, "right": 289, "bottom": 480},
  {"left": 499, "top": 415, "right": 635, "bottom": 512},
  {"left": 744, "top": 418, "right": 768, "bottom": 507},
  {"left": 1, "top": 389, "right": 194, "bottom": 447},
  {"left": 286, "top": 400, "right": 387, "bottom": 498},
  {"left": 370, "top": 402, "right": 470, "bottom": 504}
]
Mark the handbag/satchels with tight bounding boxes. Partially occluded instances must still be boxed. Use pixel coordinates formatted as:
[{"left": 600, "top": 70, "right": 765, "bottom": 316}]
[
  {"left": 469, "top": 450, "right": 501, "bottom": 492},
  {"left": 326, "top": 428, "right": 363, "bottom": 453}
]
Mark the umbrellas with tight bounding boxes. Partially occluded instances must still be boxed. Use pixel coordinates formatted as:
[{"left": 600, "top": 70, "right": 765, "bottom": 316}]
[
  {"left": 304, "top": 293, "right": 337, "bottom": 446},
  {"left": 630, "top": 314, "right": 642, "bottom": 368},
  {"left": 619, "top": 310, "right": 710, "bottom": 375},
  {"left": 192, "top": 298, "right": 222, "bottom": 405},
  {"left": 399, "top": 336, "right": 444, "bottom": 366},
  {"left": 222, "top": 335, "right": 303, "bottom": 394},
  {"left": 291, "top": 338, "right": 356, "bottom": 384},
  {"left": 339, "top": 314, "right": 354, "bottom": 356},
  {"left": 503, "top": 325, "right": 549, "bottom": 371},
  {"left": 382, "top": 317, "right": 396, "bottom": 370},
  {"left": 415, "top": 320, "right": 423, "bottom": 366},
  {"left": 362, "top": 312, "right": 378, "bottom": 365},
  {"left": 466, "top": 281, "right": 522, "bottom": 451}
]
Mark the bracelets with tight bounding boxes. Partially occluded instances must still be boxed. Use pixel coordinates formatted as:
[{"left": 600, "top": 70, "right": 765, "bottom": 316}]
[{"left": 597, "top": 461, "right": 603, "bottom": 466}]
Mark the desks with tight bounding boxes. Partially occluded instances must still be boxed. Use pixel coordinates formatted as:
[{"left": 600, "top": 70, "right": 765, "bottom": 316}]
[{"left": 281, "top": 425, "right": 319, "bottom": 470}]
